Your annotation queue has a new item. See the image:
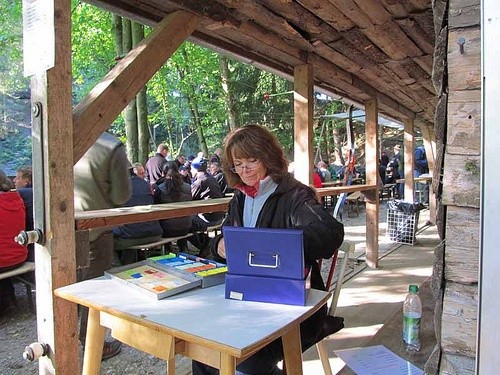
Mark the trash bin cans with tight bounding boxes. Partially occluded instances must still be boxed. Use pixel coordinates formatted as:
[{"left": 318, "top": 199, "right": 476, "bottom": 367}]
[{"left": 387, "top": 201, "right": 422, "bottom": 244}]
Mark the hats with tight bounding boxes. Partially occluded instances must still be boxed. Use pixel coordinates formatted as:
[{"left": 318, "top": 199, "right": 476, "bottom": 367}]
[{"left": 192, "top": 157, "right": 208, "bottom": 171}]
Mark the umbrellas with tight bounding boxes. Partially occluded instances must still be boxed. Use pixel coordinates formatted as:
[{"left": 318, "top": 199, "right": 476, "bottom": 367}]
[{"left": 321, "top": 110, "right": 405, "bottom": 161}]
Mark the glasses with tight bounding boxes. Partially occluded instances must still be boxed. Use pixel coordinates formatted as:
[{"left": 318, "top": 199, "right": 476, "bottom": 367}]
[{"left": 230, "top": 158, "right": 262, "bottom": 174}]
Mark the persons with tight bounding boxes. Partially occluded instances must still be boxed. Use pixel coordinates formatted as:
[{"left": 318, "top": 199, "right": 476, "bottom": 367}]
[
  {"left": 192, "top": 125, "right": 344, "bottom": 375},
  {"left": 72, "top": 131, "right": 133, "bottom": 362},
  {"left": 12, "top": 167, "right": 35, "bottom": 262},
  {"left": 109, "top": 127, "right": 431, "bottom": 265},
  {"left": 0, "top": 169, "right": 28, "bottom": 325}
]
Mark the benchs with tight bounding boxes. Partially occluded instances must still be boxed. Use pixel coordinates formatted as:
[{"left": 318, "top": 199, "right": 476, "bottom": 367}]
[
  {"left": 207, "top": 224, "right": 222, "bottom": 237},
  {"left": 381, "top": 184, "right": 395, "bottom": 201},
  {"left": 115, "top": 230, "right": 203, "bottom": 261},
  {"left": 0, "top": 262, "right": 34, "bottom": 315},
  {"left": 346, "top": 191, "right": 365, "bottom": 218}
]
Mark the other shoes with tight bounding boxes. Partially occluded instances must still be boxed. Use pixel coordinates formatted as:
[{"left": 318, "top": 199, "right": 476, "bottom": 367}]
[{"left": 103, "top": 338, "right": 121, "bottom": 359}]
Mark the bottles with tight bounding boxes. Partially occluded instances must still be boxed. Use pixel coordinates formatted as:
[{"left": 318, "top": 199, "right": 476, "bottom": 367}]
[{"left": 402, "top": 285, "right": 422, "bottom": 352}]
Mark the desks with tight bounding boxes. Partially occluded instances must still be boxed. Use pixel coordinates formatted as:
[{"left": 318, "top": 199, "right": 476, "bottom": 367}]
[
  {"left": 321, "top": 178, "right": 363, "bottom": 210},
  {"left": 54, "top": 252, "right": 333, "bottom": 375}
]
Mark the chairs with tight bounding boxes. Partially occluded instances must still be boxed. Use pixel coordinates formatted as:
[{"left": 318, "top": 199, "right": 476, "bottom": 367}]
[{"left": 303, "top": 239, "right": 354, "bottom": 375}]
